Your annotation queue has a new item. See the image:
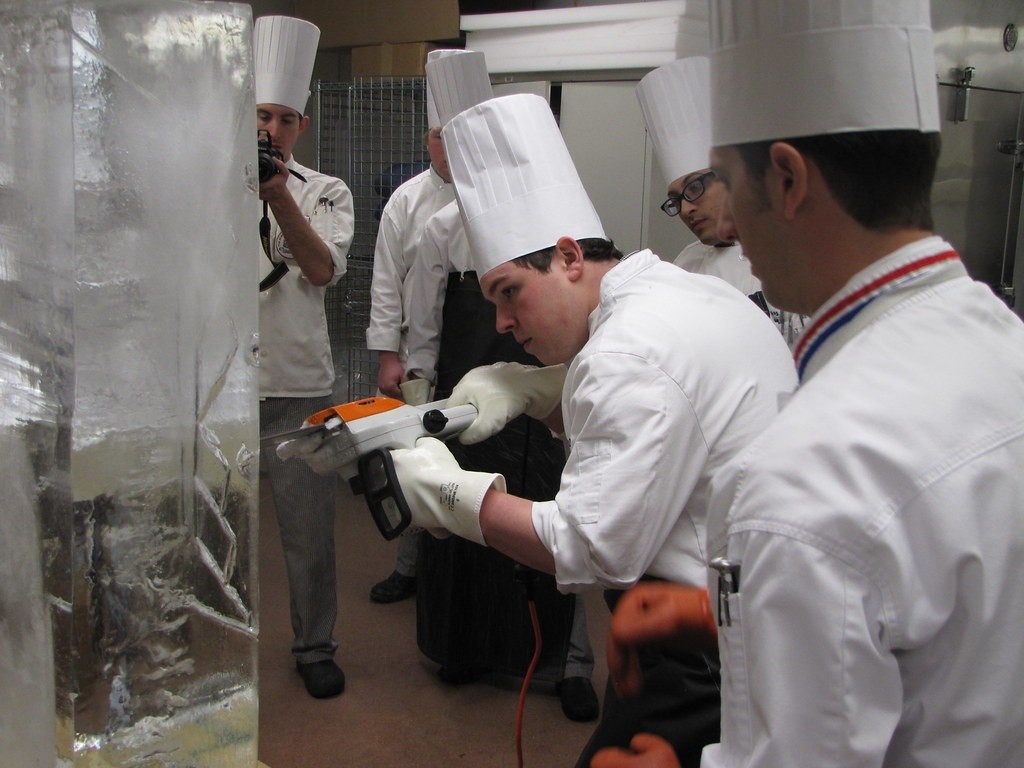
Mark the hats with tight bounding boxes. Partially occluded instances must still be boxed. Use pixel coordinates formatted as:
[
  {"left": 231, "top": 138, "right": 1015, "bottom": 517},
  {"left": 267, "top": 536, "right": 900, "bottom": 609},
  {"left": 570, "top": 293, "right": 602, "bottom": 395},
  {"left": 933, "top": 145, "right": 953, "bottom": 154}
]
[
  {"left": 440, "top": 89, "right": 610, "bottom": 279},
  {"left": 635, "top": 53, "right": 713, "bottom": 181},
  {"left": 703, "top": 0, "right": 941, "bottom": 146},
  {"left": 428, "top": 50, "right": 493, "bottom": 133},
  {"left": 254, "top": 14, "right": 322, "bottom": 114}
]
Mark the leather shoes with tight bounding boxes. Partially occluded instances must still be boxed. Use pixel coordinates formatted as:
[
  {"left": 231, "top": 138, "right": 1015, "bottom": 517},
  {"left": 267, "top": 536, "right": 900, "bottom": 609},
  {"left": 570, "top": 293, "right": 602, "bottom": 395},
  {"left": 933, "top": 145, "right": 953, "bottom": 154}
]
[{"left": 371, "top": 574, "right": 419, "bottom": 602}]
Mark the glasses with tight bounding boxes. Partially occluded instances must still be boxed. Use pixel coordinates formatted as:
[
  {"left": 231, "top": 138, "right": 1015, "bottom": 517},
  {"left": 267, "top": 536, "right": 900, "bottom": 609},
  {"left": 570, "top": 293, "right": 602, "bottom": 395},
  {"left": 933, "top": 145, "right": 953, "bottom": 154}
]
[{"left": 662, "top": 174, "right": 721, "bottom": 218}]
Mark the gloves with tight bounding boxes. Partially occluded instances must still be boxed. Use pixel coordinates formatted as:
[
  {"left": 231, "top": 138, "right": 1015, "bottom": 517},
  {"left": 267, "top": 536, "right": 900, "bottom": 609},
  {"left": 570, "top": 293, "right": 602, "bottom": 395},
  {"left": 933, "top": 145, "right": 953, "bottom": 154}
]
[
  {"left": 446, "top": 360, "right": 569, "bottom": 448},
  {"left": 375, "top": 436, "right": 507, "bottom": 547}
]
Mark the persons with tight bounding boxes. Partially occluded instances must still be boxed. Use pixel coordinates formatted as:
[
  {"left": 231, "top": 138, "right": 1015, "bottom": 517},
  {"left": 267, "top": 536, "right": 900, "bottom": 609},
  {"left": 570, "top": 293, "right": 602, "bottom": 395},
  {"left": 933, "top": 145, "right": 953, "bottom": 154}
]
[
  {"left": 254, "top": 16, "right": 357, "bottom": 702},
  {"left": 584, "top": 2, "right": 1023, "bottom": 767},
  {"left": 382, "top": 93, "right": 797, "bottom": 768},
  {"left": 633, "top": 53, "right": 815, "bottom": 354},
  {"left": 362, "top": 47, "right": 602, "bottom": 726}
]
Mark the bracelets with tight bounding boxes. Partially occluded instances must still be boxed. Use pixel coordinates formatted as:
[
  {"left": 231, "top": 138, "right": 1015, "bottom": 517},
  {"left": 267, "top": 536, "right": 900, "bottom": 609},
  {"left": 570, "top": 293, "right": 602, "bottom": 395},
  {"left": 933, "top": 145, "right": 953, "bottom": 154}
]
[{"left": 699, "top": 586, "right": 718, "bottom": 639}]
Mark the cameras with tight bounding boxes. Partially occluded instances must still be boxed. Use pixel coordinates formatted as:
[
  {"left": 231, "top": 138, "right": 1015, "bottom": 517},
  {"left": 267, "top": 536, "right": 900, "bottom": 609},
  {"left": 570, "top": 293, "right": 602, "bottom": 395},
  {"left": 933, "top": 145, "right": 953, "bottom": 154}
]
[{"left": 256, "top": 130, "right": 284, "bottom": 183}]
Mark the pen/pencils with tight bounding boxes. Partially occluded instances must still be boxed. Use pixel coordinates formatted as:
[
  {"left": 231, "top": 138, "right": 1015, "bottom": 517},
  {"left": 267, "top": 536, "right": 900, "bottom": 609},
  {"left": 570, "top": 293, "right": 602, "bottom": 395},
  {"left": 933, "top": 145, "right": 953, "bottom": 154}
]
[
  {"left": 708, "top": 557, "right": 741, "bottom": 627},
  {"left": 319, "top": 197, "right": 334, "bottom": 213}
]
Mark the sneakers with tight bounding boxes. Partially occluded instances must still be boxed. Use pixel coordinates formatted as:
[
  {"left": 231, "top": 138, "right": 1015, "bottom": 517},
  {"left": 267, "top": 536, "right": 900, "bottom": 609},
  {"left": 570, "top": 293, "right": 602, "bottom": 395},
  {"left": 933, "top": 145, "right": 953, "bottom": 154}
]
[
  {"left": 296, "top": 660, "right": 344, "bottom": 699},
  {"left": 559, "top": 675, "right": 602, "bottom": 720}
]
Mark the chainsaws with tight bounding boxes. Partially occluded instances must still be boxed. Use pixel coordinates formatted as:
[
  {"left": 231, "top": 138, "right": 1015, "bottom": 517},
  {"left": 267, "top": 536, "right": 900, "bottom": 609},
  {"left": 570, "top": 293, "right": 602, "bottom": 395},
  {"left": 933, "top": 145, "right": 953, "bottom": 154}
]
[{"left": 260, "top": 397, "right": 479, "bottom": 542}]
